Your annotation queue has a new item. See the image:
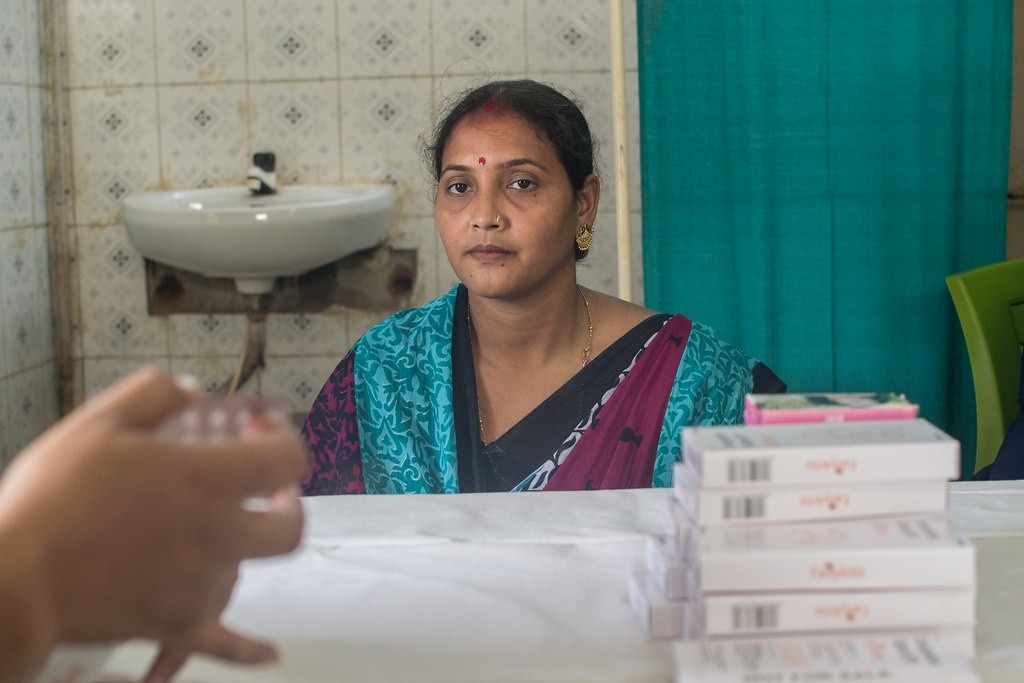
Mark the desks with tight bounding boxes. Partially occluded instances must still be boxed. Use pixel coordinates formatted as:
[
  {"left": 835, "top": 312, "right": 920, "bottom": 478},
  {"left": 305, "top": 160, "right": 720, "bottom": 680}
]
[{"left": 48, "top": 477, "right": 1024, "bottom": 683}]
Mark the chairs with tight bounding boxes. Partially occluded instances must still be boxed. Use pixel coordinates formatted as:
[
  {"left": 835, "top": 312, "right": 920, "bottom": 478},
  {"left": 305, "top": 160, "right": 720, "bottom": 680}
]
[{"left": 944, "top": 257, "right": 1024, "bottom": 473}]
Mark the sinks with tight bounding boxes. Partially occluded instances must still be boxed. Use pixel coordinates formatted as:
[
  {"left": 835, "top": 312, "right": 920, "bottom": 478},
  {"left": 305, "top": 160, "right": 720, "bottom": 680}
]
[{"left": 125, "top": 186, "right": 392, "bottom": 277}]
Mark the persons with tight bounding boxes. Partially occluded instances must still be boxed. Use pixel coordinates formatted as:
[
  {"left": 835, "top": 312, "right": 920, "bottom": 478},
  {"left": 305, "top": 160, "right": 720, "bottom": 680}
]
[
  {"left": 299, "top": 78, "right": 788, "bottom": 495},
  {"left": 0, "top": 367, "right": 304, "bottom": 683}
]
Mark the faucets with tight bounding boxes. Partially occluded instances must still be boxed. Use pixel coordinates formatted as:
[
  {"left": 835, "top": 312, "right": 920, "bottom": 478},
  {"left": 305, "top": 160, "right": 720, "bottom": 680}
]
[{"left": 246, "top": 151, "right": 277, "bottom": 193}]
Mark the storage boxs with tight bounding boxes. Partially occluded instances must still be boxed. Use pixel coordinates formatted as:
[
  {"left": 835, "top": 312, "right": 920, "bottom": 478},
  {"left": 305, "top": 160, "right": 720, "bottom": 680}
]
[{"left": 627, "top": 392, "right": 983, "bottom": 683}]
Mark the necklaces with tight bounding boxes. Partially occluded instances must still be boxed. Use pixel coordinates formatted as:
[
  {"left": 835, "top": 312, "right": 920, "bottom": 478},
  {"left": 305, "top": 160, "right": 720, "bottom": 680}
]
[{"left": 467, "top": 281, "right": 593, "bottom": 488}]
